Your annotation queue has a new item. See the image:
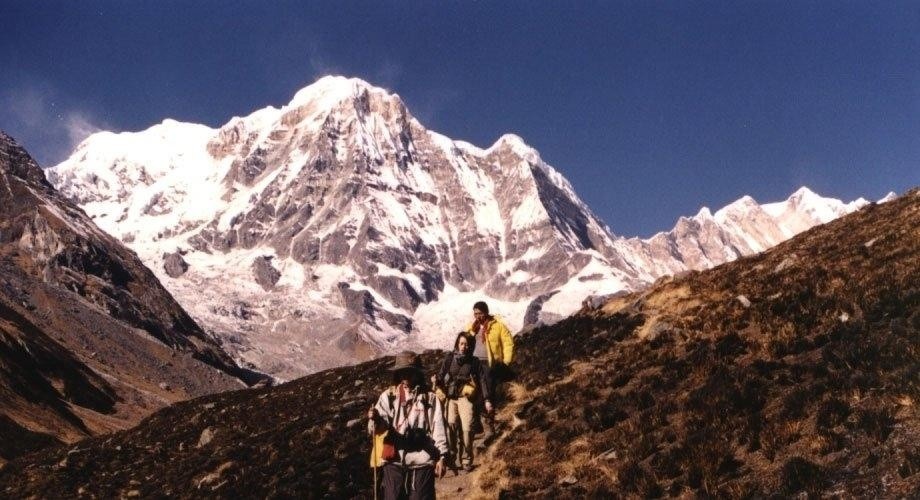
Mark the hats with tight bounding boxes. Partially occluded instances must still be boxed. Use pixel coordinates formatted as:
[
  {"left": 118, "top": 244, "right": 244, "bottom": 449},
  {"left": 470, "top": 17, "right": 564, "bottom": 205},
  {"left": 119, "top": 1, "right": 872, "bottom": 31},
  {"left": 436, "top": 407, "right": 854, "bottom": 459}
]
[{"left": 387, "top": 351, "right": 425, "bottom": 374}]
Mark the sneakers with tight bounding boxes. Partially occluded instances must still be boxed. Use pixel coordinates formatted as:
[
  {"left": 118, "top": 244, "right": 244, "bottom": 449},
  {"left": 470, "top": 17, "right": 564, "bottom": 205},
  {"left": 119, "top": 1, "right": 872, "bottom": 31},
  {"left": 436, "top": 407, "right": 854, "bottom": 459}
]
[
  {"left": 443, "top": 470, "right": 457, "bottom": 478},
  {"left": 462, "top": 464, "right": 472, "bottom": 471}
]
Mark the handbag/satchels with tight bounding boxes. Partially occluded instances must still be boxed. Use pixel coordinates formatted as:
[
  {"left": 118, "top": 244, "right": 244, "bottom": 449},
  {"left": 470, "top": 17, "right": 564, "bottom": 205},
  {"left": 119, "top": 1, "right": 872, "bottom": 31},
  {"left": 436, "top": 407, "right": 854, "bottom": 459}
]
[
  {"left": 446, "top": 381, "right": 458, "bottom": 399},
  {"left": 369, "top": 429, "right": 388, "bottom": 468}
]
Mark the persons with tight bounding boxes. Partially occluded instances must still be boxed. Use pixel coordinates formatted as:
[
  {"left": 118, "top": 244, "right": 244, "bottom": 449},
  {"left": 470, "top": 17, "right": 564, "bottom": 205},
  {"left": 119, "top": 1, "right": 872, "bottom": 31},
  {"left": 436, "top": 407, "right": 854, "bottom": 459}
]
[
  {"left": 367, "top": 351, "right": 449, "bottom": 500},
  {"left": 468, "top": 301, "right": 514, "bottom": 439},
  {"left": 431, "top": 332, "right": 494, "bottom": 471}
]
